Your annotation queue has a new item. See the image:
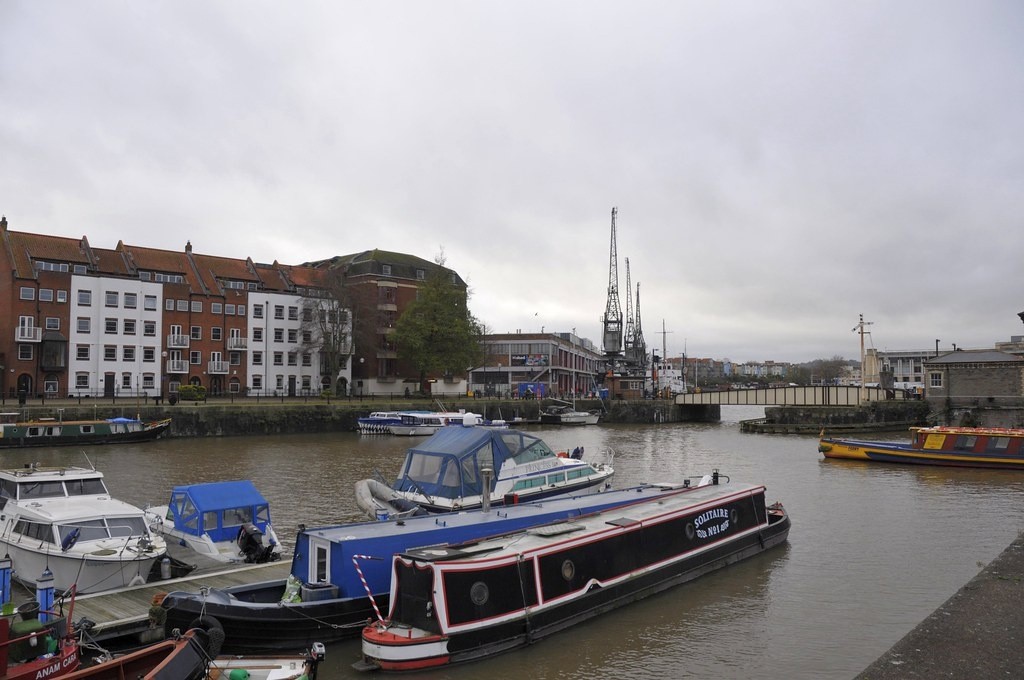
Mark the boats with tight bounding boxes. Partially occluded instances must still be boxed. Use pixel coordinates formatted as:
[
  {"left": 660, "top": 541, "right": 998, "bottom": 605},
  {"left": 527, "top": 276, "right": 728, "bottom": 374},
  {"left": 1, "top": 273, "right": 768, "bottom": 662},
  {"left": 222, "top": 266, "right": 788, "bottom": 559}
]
[
  {"left": 43, "top": 627, "right": 212, "bottom": 680},
  {"left": 157, "top": 471, "right": 717, "bottom": 660},
  {"left": 0, "top": 410, "right": 171, "bottom": 445},
  {"left": 142, "top": 479, "right": 285, "bottom": 566},
  {"left": 0, "top": 465, "right": 166, "bottom": 594},
  {"left": 357, "top": 411, "right": 510, "bottom": 435},
  {"left": 353, "top": 479, "right": 791, "bottom": 673},
  {"left": 818, "top": 425, "right": 1024, "bottom": 470},
  {"left": 395, "top": 422, "right": 613, "bottom": 512},
  {"left": 355, "top": 479, "right": 430, "bottom": 523}
]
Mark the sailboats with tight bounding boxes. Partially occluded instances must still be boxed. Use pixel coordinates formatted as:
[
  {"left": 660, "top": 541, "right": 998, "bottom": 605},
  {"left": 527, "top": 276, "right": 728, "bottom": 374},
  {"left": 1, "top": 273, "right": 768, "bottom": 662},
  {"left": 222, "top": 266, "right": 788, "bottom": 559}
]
[{"left": 538, "top": 330, "right": 601, "bottom": 425}]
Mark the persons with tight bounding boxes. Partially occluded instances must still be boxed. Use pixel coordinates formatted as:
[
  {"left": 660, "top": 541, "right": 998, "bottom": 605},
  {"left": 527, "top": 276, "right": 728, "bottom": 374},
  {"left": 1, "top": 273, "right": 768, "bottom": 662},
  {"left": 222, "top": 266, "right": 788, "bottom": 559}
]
[{"left": 470, "top": 388, "right": 593, "bottom": 401}]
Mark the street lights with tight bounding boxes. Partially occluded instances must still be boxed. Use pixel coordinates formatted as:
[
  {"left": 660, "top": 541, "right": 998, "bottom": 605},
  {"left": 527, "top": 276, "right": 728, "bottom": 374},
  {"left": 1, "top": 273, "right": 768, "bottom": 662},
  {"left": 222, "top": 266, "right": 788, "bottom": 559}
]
[
  {"left": 360, "top": 357, "right": 365, "bottom": 400},
  {"left": 161, "top": 350, "right": 167, "bottom": 403}
]
